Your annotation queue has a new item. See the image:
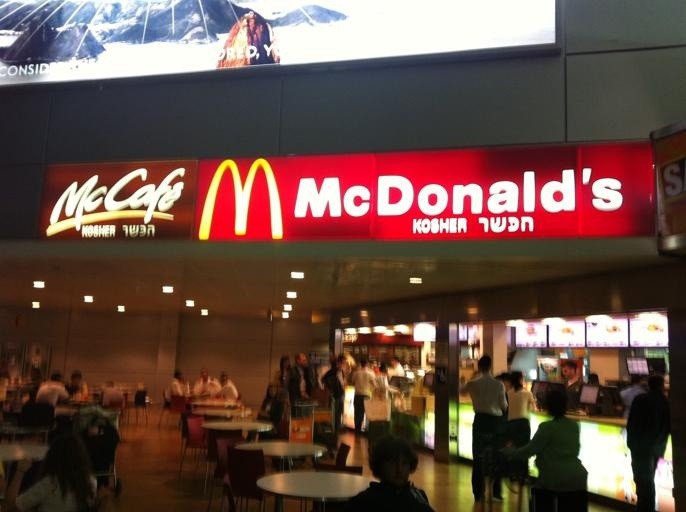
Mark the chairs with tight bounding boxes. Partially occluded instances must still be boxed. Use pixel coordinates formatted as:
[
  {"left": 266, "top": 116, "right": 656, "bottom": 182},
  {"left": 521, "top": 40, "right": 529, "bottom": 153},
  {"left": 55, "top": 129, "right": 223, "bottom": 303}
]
[
  {"left": 159, "top": 382, "right": 377, "bottom": 510},
  {"left": 2, "top": 382, "right": 150, "bottom": 508}
]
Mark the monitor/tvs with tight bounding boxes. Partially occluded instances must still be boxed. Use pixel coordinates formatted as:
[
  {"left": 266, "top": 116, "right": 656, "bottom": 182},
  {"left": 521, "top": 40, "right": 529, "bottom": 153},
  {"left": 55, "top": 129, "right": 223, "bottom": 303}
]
[
  {"left": 625, "top": 357, "right": 650, "bottom": 376},
  {"left": 602, "top": 386, "right": 621, "bottom": 416}
]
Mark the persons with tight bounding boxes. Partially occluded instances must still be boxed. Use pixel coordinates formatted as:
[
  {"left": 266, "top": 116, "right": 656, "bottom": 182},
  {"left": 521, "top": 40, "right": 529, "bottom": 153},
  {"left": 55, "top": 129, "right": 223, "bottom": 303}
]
[
  {"left": 167, "top": 368, "right": 239, "bottom": 402},
  {"left": 261, "top": 350, "right": 435, "bottom": 512},
  {"left": 1, "top": 346, "right": 148, "bottom": 511},
  {"left": 458, "top": 355, "right": 670, "bottom": 512}
]
